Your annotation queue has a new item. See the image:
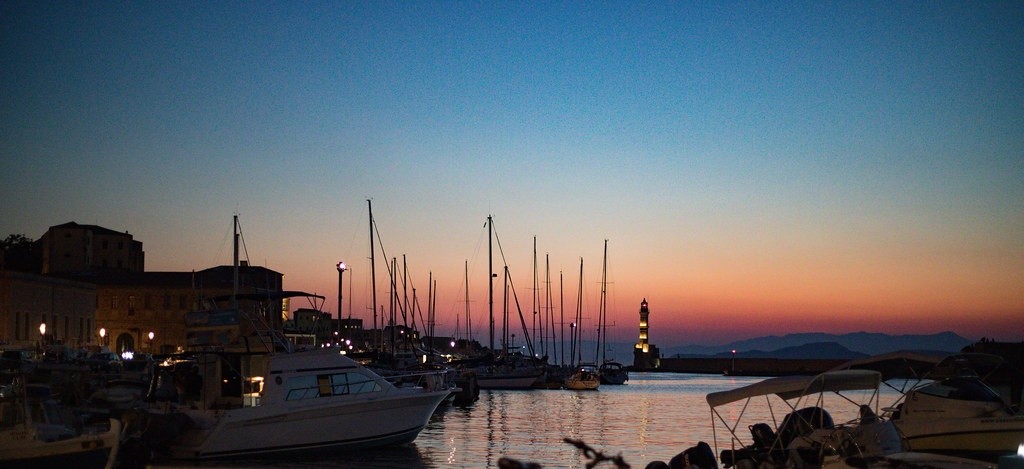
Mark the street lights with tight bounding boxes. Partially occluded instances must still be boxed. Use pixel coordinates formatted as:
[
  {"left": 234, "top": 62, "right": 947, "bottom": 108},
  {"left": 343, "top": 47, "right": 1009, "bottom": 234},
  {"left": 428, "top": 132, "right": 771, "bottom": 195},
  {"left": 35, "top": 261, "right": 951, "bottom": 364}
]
[
  {"left": 148, "top": 332, "right": 154, "bottom": 355},
  {"left": 99, "top": 328, "right": 105, "bottom": 347},
  {"left": 39, "top": 322, "right": 46, "bottom": 346}
]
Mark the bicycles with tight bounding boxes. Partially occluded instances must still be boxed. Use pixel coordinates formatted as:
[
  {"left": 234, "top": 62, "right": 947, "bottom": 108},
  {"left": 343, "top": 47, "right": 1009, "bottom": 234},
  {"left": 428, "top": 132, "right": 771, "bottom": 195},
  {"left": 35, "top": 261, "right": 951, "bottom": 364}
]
[{"left": 496, "top": 436, "right": 631, "bottom": 469}]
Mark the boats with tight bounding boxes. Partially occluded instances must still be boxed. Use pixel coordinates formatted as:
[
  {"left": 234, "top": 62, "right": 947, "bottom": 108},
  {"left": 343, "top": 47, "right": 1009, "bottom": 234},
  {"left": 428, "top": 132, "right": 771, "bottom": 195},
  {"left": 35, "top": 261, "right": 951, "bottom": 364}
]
[{"left": 705, "top": 348, "right": 1024, "bottom": 469}]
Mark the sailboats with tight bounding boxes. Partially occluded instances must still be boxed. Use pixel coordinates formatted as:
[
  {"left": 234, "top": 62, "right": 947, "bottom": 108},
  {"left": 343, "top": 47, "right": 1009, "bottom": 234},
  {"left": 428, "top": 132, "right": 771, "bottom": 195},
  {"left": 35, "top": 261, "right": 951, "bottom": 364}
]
[{"left": 0, "top": 200, "right": 630, "bottom": 469}]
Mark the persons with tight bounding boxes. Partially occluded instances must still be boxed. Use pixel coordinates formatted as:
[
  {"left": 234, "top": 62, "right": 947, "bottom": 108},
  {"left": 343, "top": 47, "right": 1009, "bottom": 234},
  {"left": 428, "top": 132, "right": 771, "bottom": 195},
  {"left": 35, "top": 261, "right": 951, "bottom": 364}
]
[{"left": 185, "top": 366, "right": 203, "bottom": 401}]
